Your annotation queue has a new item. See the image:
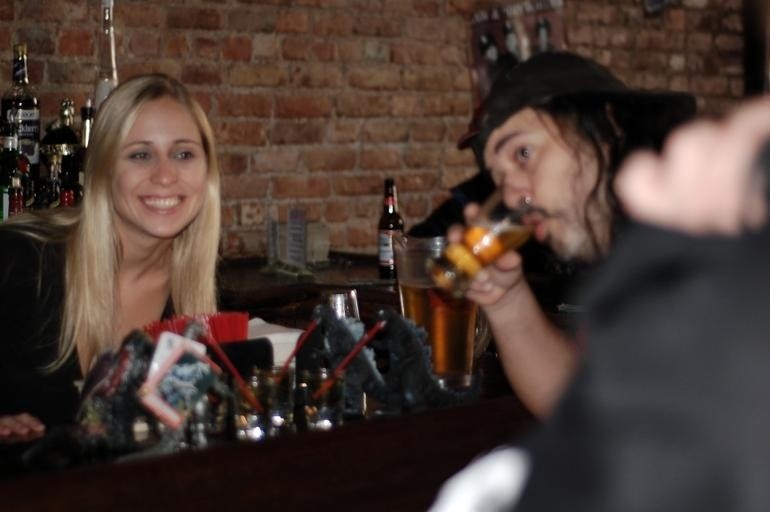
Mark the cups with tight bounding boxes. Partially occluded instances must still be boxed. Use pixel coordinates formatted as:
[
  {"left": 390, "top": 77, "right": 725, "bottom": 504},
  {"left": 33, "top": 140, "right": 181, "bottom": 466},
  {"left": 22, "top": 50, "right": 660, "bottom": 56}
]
[
  {"left": 394, "top": 187, "right": 535, "bottom": 390},
  {"left": 231, "top": 369, "right": 349, "bottom": 440},
  {"left": 321, "top": 289, "right": 369, "bottom": 418}
]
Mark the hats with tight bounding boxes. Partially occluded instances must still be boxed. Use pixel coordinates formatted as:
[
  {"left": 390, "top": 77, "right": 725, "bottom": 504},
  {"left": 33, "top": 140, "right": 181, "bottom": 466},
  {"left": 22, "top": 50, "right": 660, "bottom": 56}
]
[{"left": 457, "top": 52, "right": 699, "bottom": 151}]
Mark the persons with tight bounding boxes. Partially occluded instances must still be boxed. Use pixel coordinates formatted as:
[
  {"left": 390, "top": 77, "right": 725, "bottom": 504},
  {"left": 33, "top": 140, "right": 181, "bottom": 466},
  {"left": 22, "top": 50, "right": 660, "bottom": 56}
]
[
  {"left": 0, "top": 73, "right": 221, "bottom": 436},
  {"left": 447, "top": 49, "right": 665, "bottom": 427},
  {"left": 506, "top": 95, "right": 770, "bottom": 512}
]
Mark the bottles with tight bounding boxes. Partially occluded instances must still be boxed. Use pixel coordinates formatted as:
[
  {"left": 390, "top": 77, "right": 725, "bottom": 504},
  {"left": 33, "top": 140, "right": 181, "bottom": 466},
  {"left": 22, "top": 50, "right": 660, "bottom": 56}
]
[
  {"left": 378, "top": 178, "right": 404, "bottom": 278},
  {"left": 0, "top": 0, "right": 123, "bottom": 217}
]
